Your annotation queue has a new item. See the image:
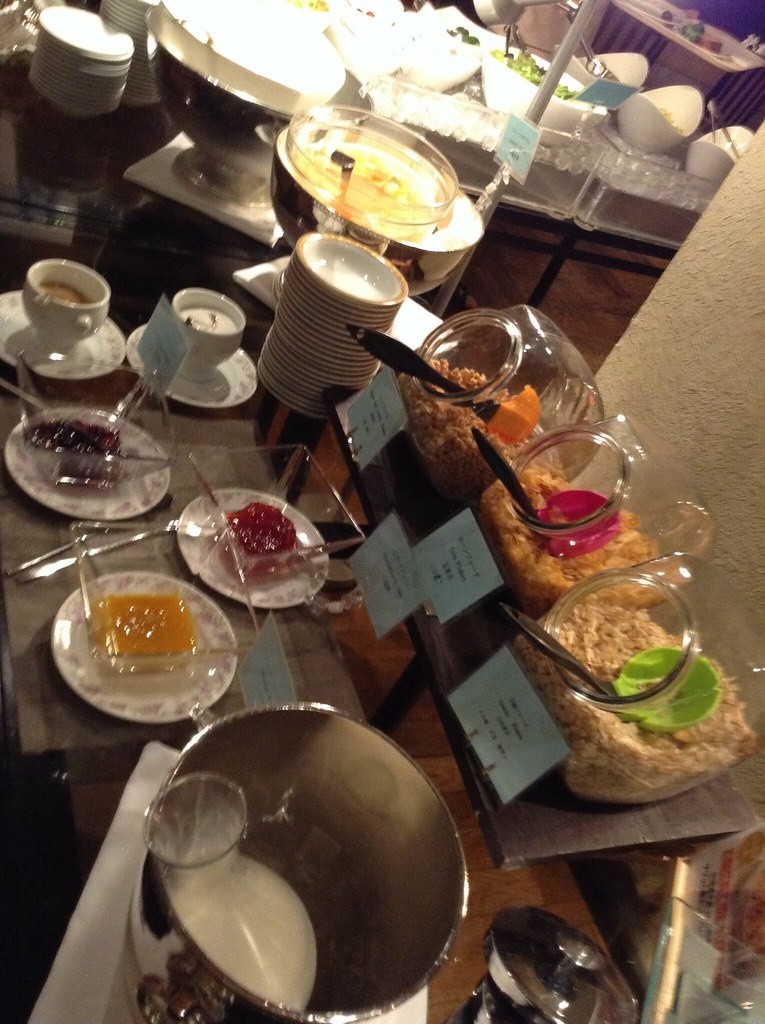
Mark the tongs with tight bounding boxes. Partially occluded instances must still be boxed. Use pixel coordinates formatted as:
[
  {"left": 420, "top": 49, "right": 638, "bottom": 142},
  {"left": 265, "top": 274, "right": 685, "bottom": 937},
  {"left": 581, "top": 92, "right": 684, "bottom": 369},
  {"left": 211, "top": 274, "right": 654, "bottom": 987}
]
[{"left": 707, "top": 99, "right": 739, "bottom": 158}]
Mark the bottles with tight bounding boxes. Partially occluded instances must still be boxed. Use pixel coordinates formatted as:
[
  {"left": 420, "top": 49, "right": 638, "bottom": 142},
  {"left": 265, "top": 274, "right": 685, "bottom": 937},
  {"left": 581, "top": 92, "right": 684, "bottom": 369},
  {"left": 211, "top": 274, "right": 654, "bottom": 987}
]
[
  {"left": 515, "top": 550, "right": 765, "bottom": 805},
  {"left": 478, "top": 414, "right": 713, "bottom": 613},
  {"left": 398, "top": 303, "right": 605, "bottom": 500},
  {"left": 143, "top": 773, "right": 316, "bottom": 1013}
]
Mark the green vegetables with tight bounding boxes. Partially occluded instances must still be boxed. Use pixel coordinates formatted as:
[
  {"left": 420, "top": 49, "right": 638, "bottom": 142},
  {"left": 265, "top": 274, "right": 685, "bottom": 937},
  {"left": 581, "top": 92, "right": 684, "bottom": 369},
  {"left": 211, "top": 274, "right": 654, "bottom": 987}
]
[{"left": 496, "top": 50, "right": 581, "bottom": 99}]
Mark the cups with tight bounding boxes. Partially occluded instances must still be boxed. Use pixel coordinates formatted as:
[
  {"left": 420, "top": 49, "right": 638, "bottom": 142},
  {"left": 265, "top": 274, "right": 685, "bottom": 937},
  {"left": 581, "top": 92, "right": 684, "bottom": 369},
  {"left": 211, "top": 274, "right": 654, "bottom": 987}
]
[
  {"left": 171, "top": 289, "right": 246, "bottom": 380},
  {"left": 23, "top": 258, "right": 112, "bottom": 345},
  {"left": 129, "top": 703, "right": 471, "bottom": 1024}
]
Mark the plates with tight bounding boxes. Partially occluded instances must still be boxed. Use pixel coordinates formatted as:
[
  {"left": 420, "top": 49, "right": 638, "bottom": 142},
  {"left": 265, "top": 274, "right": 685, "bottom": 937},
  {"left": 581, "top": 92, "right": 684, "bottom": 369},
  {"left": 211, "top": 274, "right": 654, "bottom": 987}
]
[
  {"left": 176, "top": 488, "right": 329, "bottom": 609},
  {"left": 50, "top": 572, "right": 239, "bottom": 724},
  {"left": 0, "top": 290, "right": 126, "bottom": 380},
  {"left": 4, "top": 406, "right": 171, "bottom": 520},
  {"left": 126, "top": 323, "right": 258, "bottom": 409},
  {"left": 621, "top": 0, "right": 685, "bottom": 26},
  {"left": 671, "top": 23, "right": 744, "bottom": 59},
  {"left": 257, "top": 231, "right": 409, "bottom": 420},
  {"left": 29, "top": 0, "right": 162, "bottom": 120}
]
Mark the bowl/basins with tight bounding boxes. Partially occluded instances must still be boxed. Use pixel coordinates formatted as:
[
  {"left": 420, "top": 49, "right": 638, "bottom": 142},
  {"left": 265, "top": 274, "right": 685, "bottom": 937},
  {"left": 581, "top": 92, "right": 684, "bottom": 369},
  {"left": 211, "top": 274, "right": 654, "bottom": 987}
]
[
  {"left": 390, "top": 10, "right": 481, "bottom": 93},
  {"left": 68, "top": 520, "right": 261, "bottom": 675},
  {"left": 687, "top": 125, "right": 755, "bottom": 182},
  {"left": 266, "top": 126, "right": 484, "bottom": 298},
  {"left": 480, "top": 46, "right": 608, "bottom": 132},
  {"left": 617, "top": 85, "right": 703, "bottom": 153},
  {"left": 142, "top": 6, "right": 295, "bottom": 204},
  {"left": 286, "top": 104, "right": 459, "bottom": 242},
  {"left": 566, "top": 52, "right": 649, "bottom": 89},
  {"left": 640, "top": 895, "right": 765, "bottom": 1024},
  {"left": 15, "top": 349, "right": 178, "bottom": 495},
  {"left": 186, "top": 442, "right": 366, "bottom": 585}
]
